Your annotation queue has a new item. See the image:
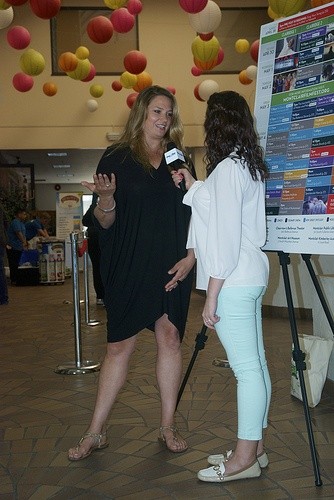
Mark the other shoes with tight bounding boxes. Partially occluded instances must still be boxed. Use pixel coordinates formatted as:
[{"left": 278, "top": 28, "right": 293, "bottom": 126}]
[
  {"left": 207, "top": 449, "right": 269, "bottom": 468},
  {"left": 197, "top": 455, "right": 261, "bottom": 481}
]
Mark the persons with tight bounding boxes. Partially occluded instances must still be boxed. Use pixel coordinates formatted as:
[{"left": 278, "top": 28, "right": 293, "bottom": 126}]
[
  {"left": 171, "top": 90, "right": 272, "bottom": 483},
  {"left": 87, "top": 233, "right": 105, "bottom": 307},
  {"left": 68, "top": 85, "right": 197, "bottom": 459},
  {"left": 6, "top": 209, "right": 51, "bottom": 286},
  {"left": 0, "top": 247, "right": 8, "bottom": 305},
  {"left": 278, "top": 38, "right": 294, "bottom": 57},
  {"left": 273, "top": 72, "right": 296, "bottom": 94}
]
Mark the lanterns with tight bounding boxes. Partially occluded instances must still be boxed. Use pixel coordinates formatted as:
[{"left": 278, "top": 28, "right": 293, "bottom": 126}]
[
  {"left": 195, "top": 80, "right": 219, "bottom": 101},
  {"left": 167, "top": 86, "right": 176, "bottom": 95},
  {"left": 7, "top": 25, "right": 57, "bottom": 97},
  {"left": 0, "top": 0, "right": 61, "bottom": 31},
  {"left": 112, "top": 50, "right": 152, "bottom": 109},
  {"left": 87, "top": 0, "right": 142, "bottom": 44},
  {"left": 235, "top": 39, "right": 260, "bottom": 85},
  {"left": 58, "top": 45, "right": 103, "bottom": 112},
  {"left": 178, "top": 0, "right": 224, "bottom": 75},
  {"left": 266, "top": 0, "right": 334, "bottom": 21}
]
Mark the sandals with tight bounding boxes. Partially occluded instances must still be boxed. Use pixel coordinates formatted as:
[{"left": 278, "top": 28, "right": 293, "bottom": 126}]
[
  {"left": 160, "top": 425, "right": 187, "bottom": 453},
  {"left": 68, "top": 431, "right": 109, "bottom": 461}
]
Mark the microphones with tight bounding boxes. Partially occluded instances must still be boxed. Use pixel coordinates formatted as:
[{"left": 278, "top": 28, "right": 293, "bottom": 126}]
[{"left": 165, "top": 142, "right": 186, "bottom": 193}]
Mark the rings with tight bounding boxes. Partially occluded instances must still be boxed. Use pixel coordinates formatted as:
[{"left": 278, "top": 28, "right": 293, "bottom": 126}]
[
  {"left": 177, "top": 280, "right": 181, "bottom": 284},
  {"left": 106, "top": 185, "right": 110, "bottom": 187}
]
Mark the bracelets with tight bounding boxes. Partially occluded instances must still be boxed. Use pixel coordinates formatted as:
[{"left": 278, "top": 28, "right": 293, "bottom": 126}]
[{"left": 96, "top": 197, "right": 116, "bottom": 212}]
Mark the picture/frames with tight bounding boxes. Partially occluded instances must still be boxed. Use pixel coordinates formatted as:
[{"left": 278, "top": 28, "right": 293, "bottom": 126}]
[
  {"left": 50, "top": 5, "right": 140, "bottom": 76},
  {"left": 196, "top": 7, "right": 274, "bottom": 75}
]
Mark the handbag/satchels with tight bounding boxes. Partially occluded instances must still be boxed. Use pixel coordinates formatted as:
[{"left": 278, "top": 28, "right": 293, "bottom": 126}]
[{"left": 291, "top": 333, "right": 334, "bottom": 408}]
